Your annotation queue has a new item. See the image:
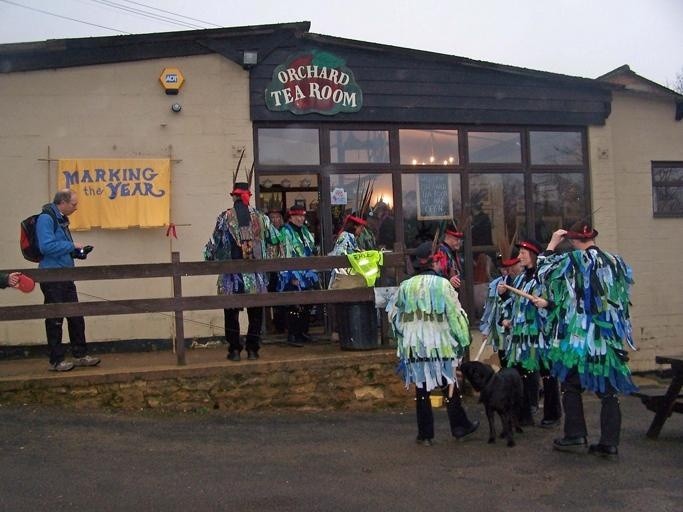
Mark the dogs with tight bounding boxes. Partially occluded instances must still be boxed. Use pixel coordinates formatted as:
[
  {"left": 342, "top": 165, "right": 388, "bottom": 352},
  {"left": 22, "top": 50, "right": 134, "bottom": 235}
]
[{"left": 459, "top": 361, "right": 526, "bottom": 447}]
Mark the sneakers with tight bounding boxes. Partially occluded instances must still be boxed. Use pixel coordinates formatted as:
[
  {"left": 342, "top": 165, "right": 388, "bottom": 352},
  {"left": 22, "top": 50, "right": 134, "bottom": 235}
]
[
  {"left": 47, "top": 359, "right": 74, "bottom": 372},
  {"left": 72, "top": 355, "right": 101, "bottom": 366}
]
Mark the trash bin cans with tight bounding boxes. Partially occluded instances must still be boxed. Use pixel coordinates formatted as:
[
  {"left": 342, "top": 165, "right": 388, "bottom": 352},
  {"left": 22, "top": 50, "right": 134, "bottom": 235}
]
[{"left": 335, "top": 301, "right": 378, "bottom": 349}]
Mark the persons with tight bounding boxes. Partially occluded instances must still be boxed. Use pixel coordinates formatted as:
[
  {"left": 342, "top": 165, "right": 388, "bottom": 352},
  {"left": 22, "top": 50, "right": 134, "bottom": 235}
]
[
  {"left": 384, "top": 248, "right": 479, "bottom": 446},
  {"left": 35, "top": 187, "right": 102, "bottom": 372},
  {"left": 265, "top": 199, "right": 492, "bottom": 351},
  {"left": 0, "top": 270, "right": 22, "bottom": 289},
  {"left": 479, "top": 221, "right": 640, "bottom": 461},
  {"left": 204, "top": 179, "right": 282, "bottom": 360}
]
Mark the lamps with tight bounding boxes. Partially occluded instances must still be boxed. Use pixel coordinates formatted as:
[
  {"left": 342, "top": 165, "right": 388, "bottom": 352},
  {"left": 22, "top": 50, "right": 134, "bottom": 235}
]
[{"left": 409, "top": 129, "right": 455, "bottom": 165}]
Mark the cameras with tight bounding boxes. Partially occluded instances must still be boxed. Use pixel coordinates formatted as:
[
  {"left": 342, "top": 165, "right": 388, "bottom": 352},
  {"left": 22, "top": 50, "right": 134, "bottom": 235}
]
[{"left": 69, "top": 245, "right": 94, "bottom": 261}]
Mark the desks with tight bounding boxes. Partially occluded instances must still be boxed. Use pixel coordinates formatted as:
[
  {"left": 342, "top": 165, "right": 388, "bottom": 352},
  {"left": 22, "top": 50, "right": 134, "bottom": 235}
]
[{"left": 639, "top": 352, "right": 682, "bottom": 443}]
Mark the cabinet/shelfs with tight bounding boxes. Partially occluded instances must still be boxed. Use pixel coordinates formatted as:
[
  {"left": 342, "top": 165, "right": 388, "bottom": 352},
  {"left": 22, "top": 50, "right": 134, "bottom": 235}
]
[{"left": 258, "top": 184, "right": 321, "bottom": 245}]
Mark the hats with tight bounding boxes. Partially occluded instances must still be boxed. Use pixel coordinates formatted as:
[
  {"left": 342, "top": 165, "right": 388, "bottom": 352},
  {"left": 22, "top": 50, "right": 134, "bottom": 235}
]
[
  {"left": 515, "top": 238, "right": 543, "bottom": 256},
  {"left": 444, "top": 224, "right": 464, "bottom": 238},
  {"left": 287, "top": 204, "right": 307, "bottom": 216},
  {"left": 267, "top": 207, "right": 283, "bottom": 214},
  {"left": 348, "top": 211, "right": 368, "bottom": 226},
  {"left": 408, "top": 241, "right": 441, "bottom": 269},
  {"left": 230, "top": 182, "right": 253, "bottom": 197},
  {"left": 496, "top": 247, "right": 521, "bottom": 268},
  {"left": 561, "top": 220, "right": 598, "bottom": 240}
]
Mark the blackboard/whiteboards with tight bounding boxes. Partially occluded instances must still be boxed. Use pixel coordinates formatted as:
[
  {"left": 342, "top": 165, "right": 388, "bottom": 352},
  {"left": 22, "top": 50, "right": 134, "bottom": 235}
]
[{"left": 417, "top": 174, "right": 452, "bottom": 220}]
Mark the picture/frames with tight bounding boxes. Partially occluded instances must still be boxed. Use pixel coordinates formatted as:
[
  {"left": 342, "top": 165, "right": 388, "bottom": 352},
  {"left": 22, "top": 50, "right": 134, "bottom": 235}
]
[
  {"left": 467, "top": 184, "right": 491, "bottom": 207},
  {"left": 479, "top": 208, "right": 493, "bottom": 228}
]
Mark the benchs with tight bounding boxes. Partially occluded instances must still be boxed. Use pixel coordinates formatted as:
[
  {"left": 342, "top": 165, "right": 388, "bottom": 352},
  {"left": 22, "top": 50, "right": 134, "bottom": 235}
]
[{"left": 627, "top": 384, "right": 682, "bottom": 417}]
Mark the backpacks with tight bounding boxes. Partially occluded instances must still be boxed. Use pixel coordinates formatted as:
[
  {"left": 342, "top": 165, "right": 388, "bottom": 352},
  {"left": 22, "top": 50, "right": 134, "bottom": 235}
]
[{"left": 19, "top": 209, "right": 58, "bottom": 264}]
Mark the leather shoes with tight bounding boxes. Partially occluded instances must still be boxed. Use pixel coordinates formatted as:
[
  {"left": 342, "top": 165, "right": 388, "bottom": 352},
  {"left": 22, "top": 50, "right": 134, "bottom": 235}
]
[
  {"left": 416, "top": 435, "right": 433, "bottom": 447},
  {"left": 452, "top": 419, "right": 481, "bottom": 443},
  {"left": 285, "top": 330, "right": 320, "bottom": 347},
  {"left": 227, "top": 349, "right": 240, "bottom": 362},
  {"left": 247, "top": 351, "right": 258, "bottom": 360},
  {"left": 553, "top": 436, "right": 589, "bottom": 455},
  {"left": 537, "top": 417, "right": 561, "bottom": 429},
  {"left": 530, "top": 405, "right": 541, "bottom": 416},
  {"left": 587, "top": 443, "right": 620, "bottom": 462}
]
